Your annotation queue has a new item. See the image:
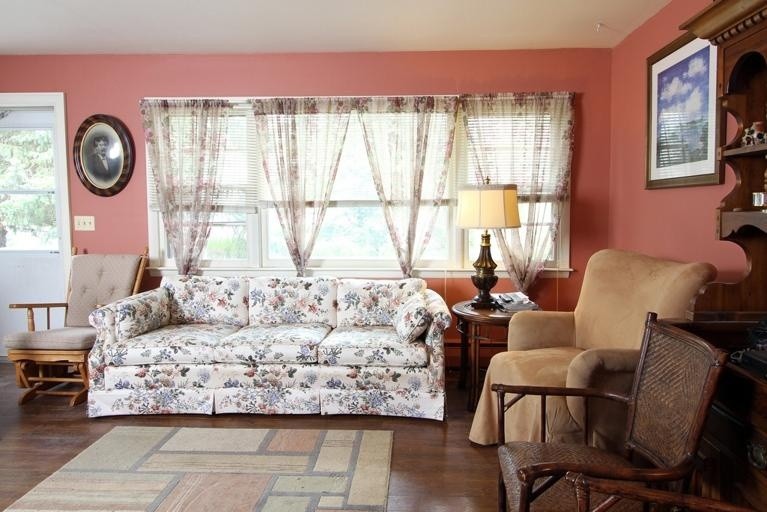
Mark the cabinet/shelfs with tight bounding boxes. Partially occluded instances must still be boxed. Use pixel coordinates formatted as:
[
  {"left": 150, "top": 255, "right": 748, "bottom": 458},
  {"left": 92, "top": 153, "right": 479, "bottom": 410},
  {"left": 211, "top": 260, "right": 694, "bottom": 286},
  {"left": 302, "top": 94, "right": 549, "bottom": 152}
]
[{"left": 659, "top": 0, "right": 767, "bottom": 512}]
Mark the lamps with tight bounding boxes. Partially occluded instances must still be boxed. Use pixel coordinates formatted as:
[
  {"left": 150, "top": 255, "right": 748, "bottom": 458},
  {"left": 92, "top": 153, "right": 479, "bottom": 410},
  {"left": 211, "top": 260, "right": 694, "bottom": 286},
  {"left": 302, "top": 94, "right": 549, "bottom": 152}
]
[{"left": 455, "top": 175, "right": 521, "bottom": 310}]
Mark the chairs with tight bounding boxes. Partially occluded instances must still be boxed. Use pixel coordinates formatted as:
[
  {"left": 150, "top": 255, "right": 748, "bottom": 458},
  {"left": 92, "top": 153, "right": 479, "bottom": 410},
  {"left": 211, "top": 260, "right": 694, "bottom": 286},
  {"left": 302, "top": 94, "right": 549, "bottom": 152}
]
[
  {"left": 563, "top": 471, "right": 754, "bottom": 512},
  {"left": 488, "top": 310, "right": 731, "bottom": 512},
  {"left": 7, "top": 246, "right": 150, "bottom": 407}
]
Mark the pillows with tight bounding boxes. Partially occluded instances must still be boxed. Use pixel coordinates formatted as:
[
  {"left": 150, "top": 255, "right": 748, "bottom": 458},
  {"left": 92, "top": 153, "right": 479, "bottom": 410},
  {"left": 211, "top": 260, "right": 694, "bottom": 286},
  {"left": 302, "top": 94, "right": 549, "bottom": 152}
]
[
  {"left": 114, "top": 288, "right": 171, "bottom": 342},
  {"left": 389, "top": 292, "right": 432, "bottom": 343}
]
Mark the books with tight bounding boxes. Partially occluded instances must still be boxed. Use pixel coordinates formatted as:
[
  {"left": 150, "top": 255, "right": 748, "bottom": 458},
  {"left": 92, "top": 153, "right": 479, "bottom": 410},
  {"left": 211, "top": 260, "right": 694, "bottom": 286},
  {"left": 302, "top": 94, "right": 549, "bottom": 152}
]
[{"left": 490, "top": 291, "right": 539, "bottom": 313}]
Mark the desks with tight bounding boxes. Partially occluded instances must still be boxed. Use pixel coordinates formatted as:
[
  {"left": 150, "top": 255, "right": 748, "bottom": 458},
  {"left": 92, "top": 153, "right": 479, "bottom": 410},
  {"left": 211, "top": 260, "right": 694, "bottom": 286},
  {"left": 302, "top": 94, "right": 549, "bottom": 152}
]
[{"left": 452, "top": 296, "right": 539, "bottom": 413}]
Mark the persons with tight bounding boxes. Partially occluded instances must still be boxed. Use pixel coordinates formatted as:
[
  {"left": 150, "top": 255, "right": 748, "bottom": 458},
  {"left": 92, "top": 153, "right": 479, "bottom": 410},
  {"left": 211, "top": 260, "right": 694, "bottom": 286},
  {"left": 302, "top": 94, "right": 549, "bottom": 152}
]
[{"left": 87, "top": 135, "right": 118, "bottom": 182}]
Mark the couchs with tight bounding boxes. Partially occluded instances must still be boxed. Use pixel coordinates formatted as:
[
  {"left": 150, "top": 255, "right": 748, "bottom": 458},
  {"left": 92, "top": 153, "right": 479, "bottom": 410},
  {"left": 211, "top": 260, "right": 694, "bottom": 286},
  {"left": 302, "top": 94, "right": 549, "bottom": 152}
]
[
  {"left": 85, "top": 272, "right": 454, "bottom": 424},
  {"left": 470, "top": 248, "right": 718, "bottom": 448}
]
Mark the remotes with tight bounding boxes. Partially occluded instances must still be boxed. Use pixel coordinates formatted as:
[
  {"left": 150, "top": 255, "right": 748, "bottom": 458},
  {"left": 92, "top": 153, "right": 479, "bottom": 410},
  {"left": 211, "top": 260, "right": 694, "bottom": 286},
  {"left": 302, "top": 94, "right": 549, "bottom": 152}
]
[
  {"left": 499, "top": 294, "right": 512, "bottom": 303},
  {"left": 509, "top": 294, "right": 523, "bottom": 303}
]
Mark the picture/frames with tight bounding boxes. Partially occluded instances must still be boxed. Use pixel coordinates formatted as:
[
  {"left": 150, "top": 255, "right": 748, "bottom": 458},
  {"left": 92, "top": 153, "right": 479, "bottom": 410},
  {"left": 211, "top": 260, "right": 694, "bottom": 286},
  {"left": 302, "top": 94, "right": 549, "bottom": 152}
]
[
  {"left": 644, "top": 31, "right": 726, "bottom": 191},
  {"left": 73, "top": 113, "right": 134, "bottom": 197}
]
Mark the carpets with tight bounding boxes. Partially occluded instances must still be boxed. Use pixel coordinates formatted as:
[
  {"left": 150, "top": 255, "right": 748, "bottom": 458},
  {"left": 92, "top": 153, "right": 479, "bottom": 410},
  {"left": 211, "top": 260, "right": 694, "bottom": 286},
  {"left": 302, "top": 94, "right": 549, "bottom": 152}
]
[{"left": 2, "top": 427, "right": 393, "bottom": 512}]
